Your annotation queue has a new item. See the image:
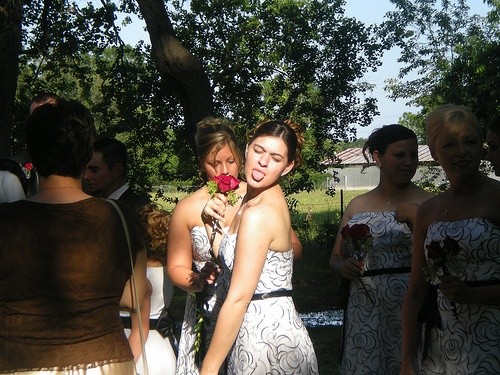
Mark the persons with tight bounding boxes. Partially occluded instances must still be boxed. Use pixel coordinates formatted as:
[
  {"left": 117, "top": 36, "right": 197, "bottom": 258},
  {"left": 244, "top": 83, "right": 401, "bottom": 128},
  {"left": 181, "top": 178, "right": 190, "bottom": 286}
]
[
  {"left": 328, "top": 124, "right": 439, "bottom": 375},
  {"left": 201, "top": 119, "right": 318, "bottom": 375},
  {"left": 401, "top": 104, "right": 500, "bottom": 374},
  {"left": 0, "top": 92, "right": 181, "bottom": 375},
  {"left": 165, "top": 121, "right": 303, "bottom": 375}
]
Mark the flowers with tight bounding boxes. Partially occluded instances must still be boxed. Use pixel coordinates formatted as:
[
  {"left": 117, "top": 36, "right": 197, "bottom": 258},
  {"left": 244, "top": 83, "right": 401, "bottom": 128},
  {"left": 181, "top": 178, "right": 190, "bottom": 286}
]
[
  {"left": 205, "top": 174, "right": 243, "bottom": 232},
  {"left": 424, "top": 236, "right": 464, "bottom": 320},
  {"left": 340, "top": 223, "right": 377, "bottom": 306}
]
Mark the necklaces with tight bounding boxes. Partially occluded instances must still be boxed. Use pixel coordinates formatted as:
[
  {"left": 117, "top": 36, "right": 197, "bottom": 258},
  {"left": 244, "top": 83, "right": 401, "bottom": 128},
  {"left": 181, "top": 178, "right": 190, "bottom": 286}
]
[
  {"left": 385, "top": 201, "right": 392, "bottom": 205},
  {"left": 44, "top": 187, "right": 80, "bottom": 190},
  {"left": 238, "top": 190, "right": 258, "bottom": 215}
]
